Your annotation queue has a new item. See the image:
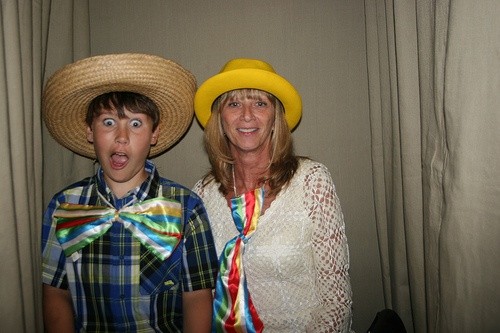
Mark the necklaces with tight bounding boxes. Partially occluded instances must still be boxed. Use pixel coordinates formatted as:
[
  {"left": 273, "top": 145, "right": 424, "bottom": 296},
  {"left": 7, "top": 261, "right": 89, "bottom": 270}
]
[{"left": 231, "top": 164, "right": 268, "bottom": 197}]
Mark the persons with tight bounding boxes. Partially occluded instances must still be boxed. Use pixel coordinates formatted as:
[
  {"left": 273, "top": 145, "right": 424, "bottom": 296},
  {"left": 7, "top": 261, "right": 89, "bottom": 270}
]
[
  {"left": 191, "top": 59, "right": 351, "bottom": 333},
  {"left": 41, "top": 53, "right": 218, "bottom": 333}
]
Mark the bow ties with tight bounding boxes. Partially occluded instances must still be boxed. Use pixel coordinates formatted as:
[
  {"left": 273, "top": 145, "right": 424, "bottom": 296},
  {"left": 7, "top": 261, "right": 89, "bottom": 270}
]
[{"left": 53, "top": 196, "right": 183, "bottom": 261}]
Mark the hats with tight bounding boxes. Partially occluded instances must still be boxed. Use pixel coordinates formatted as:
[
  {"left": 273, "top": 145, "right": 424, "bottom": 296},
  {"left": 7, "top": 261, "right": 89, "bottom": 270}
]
[
  {"left": 193, "top": 59, "right": 302, "bottom": 131},
  {"left": 41, "top": 53, "right": 198, "bottom": 160}
]
